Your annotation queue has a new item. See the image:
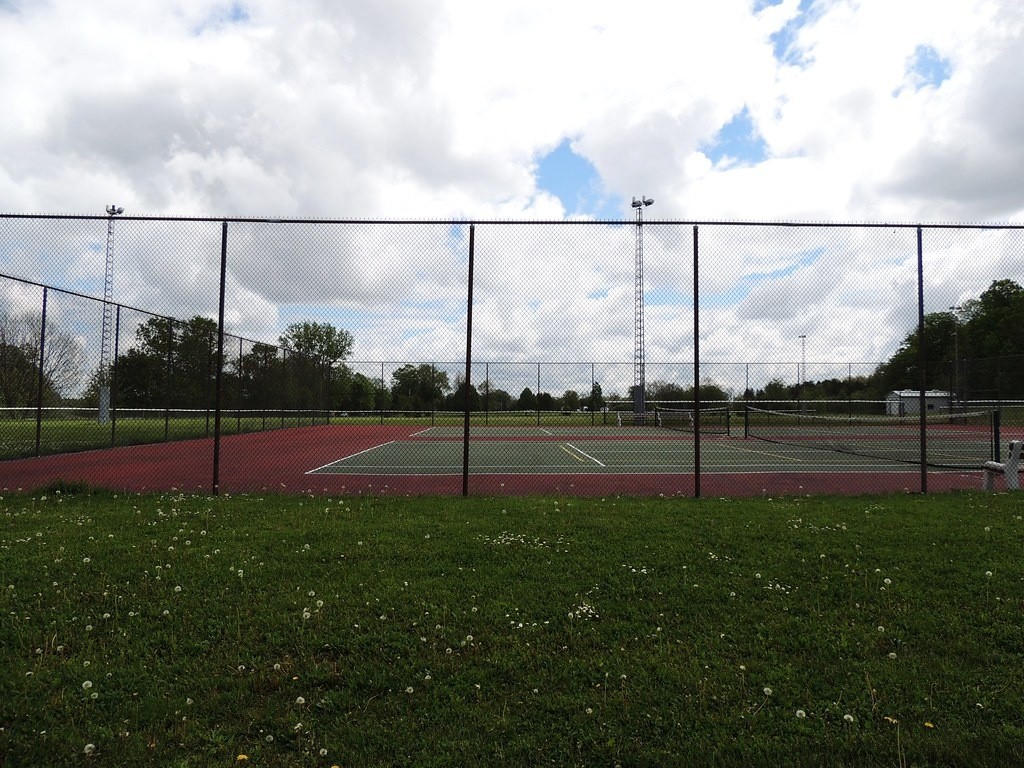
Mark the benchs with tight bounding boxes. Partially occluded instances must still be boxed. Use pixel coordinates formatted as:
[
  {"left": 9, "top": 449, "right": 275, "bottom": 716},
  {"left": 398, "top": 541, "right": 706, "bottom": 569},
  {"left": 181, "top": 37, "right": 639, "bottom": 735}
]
[{"left": 983, "top": 440, "right": 1024, "bottom": 492}]
